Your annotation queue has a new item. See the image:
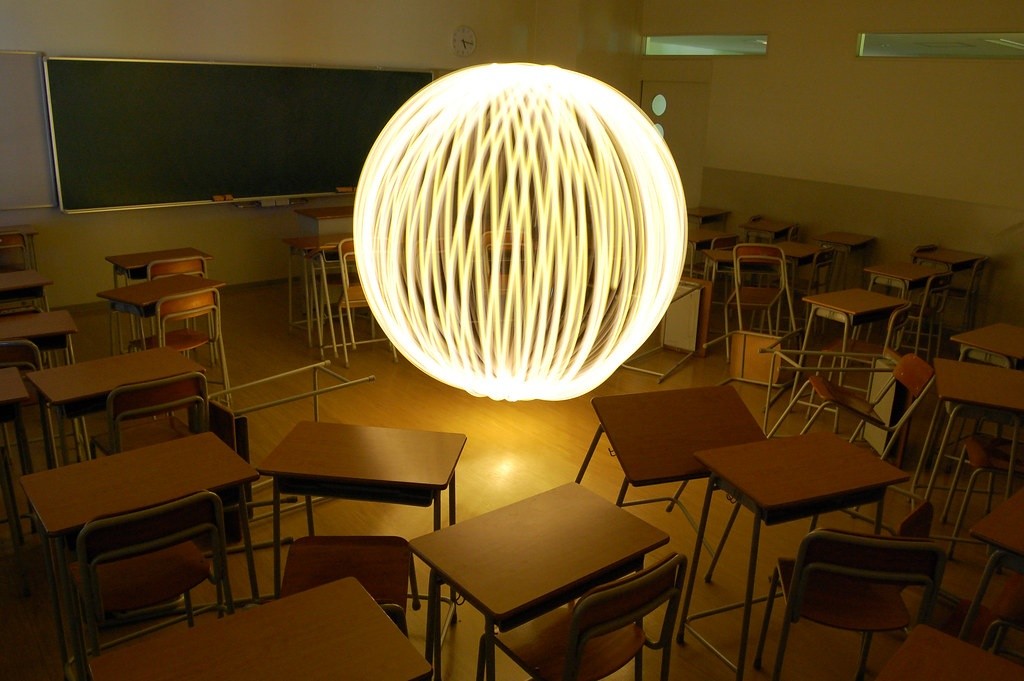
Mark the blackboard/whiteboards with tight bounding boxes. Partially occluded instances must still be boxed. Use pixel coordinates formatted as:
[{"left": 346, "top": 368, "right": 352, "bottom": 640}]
[
  {"left": 43, "top": 56, "right": 436, "bottom": 216},
  {"left": 0, "top": 50, "right": 59, "bottom": 211}
]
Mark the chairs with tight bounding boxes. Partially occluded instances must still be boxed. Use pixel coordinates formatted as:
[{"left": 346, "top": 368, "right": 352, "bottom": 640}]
[
  {"left": 476, "top": 551, "right": 690, "bottom": 681},
  {"left": 0, "top": 230, "right": 1022, "bottom": 681},
  {"left": 755, "top": 526, "right": 947, "bottom": 680}
]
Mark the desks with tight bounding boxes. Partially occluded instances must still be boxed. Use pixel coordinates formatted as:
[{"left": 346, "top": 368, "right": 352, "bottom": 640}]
[
  {"left": 872, "top": 626, "right": 1022, "bottom": 681},
  {"left": 675, "top": 432, "right": 912, "bottom": 680},
  {"left": 409, "top": 482, "right": 671, "bottom": 681},
  {"left": 0, "top": 214, "right": 1023, "bottom": 606},
  {"left": 85, "top": 577, "right": 435, "bottom": 681},
  {"left": 20, "top": 431, "right": 260, "bottom": 679}
]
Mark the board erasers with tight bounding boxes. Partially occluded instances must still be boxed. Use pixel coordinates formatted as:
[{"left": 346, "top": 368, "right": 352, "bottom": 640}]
[
  {"left": 336, "top": 187, "right": 357, "bottom": 192},
  {"left": 212, "top": 195, "right": 233, "bottom": 201}
]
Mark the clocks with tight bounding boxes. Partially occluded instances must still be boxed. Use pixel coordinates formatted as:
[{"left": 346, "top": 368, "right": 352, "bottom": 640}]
[{"left": 451, "top": 25, "right": 477, "bottom": 58}]
